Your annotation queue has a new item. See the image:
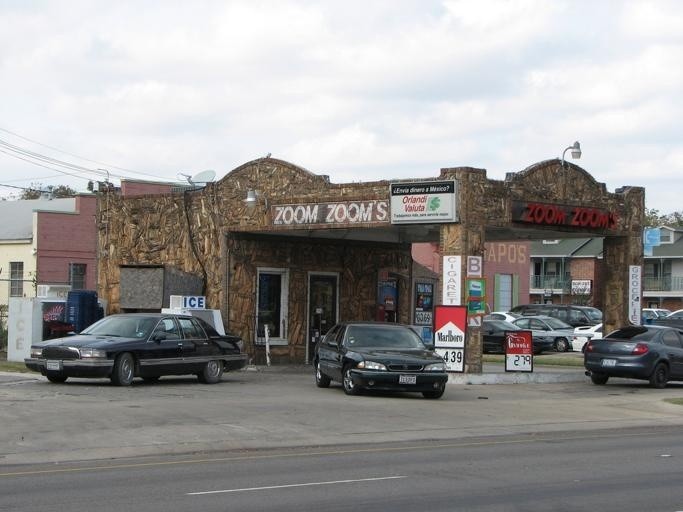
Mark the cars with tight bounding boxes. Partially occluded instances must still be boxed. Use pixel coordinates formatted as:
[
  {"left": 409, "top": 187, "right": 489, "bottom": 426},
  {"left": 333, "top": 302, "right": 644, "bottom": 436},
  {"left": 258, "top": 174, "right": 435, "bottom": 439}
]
[
  {"left": 481, "top": 304, "right": 683, "bottom": 354},
  {"left": 313, "top": 321, "right": 449, "bottom": 398},
  {"left": 583, "top": 325, "right": 682, "bottom": 389},
  {"left": 24, "top": 313, "right": 248, "bottom": 387}
]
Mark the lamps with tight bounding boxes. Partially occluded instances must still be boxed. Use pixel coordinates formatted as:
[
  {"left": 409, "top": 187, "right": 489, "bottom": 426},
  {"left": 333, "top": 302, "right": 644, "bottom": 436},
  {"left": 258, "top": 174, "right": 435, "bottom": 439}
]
[
  {"left": 561, "top": 140, "right": 581, "bottom": 167},
  {"left": 245, "top": 187, "right": 257, "bottom": 202}
]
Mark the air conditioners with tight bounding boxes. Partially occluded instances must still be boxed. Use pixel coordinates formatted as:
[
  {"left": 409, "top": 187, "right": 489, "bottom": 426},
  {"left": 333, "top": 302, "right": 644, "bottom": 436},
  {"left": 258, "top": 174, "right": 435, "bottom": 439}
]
[{"left": 36, "top": 284, "right": 71, "bottom": 299}]
